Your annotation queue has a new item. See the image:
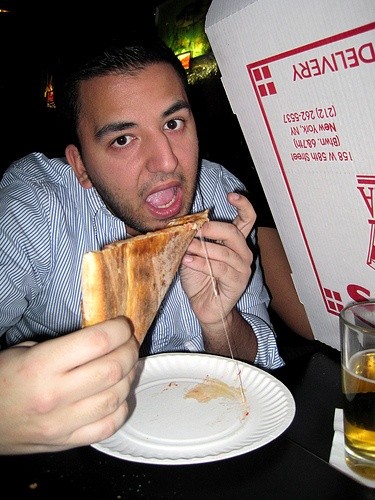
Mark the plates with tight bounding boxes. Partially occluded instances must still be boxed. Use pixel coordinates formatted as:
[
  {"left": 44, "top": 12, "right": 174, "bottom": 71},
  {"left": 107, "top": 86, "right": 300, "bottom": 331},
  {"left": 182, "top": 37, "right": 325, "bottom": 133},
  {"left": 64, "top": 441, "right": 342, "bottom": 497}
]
[{"left": 88, "top": 352, "right": 296, "bottom": 465}]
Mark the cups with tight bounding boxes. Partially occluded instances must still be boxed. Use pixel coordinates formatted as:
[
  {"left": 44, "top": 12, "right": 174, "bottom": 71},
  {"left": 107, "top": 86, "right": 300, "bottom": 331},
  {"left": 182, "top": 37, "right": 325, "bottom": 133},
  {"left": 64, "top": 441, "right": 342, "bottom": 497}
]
[{"left": 338, "top": 298, "right": 375, "bottom": 481}]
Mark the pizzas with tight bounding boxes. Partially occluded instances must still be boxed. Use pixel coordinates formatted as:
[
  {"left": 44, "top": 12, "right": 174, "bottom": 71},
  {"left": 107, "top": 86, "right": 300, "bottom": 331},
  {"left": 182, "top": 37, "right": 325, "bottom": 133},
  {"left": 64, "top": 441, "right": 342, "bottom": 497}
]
[{"left": 81, "top": 210, "right": 208, "bottom": 352}]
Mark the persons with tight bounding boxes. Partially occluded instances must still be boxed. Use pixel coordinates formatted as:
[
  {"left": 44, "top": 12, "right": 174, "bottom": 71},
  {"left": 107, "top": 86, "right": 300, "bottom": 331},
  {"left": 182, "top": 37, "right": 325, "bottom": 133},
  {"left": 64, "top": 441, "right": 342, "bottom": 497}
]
[{"left": 1, "top": 27, "right": 287, "bottom": 453}]
